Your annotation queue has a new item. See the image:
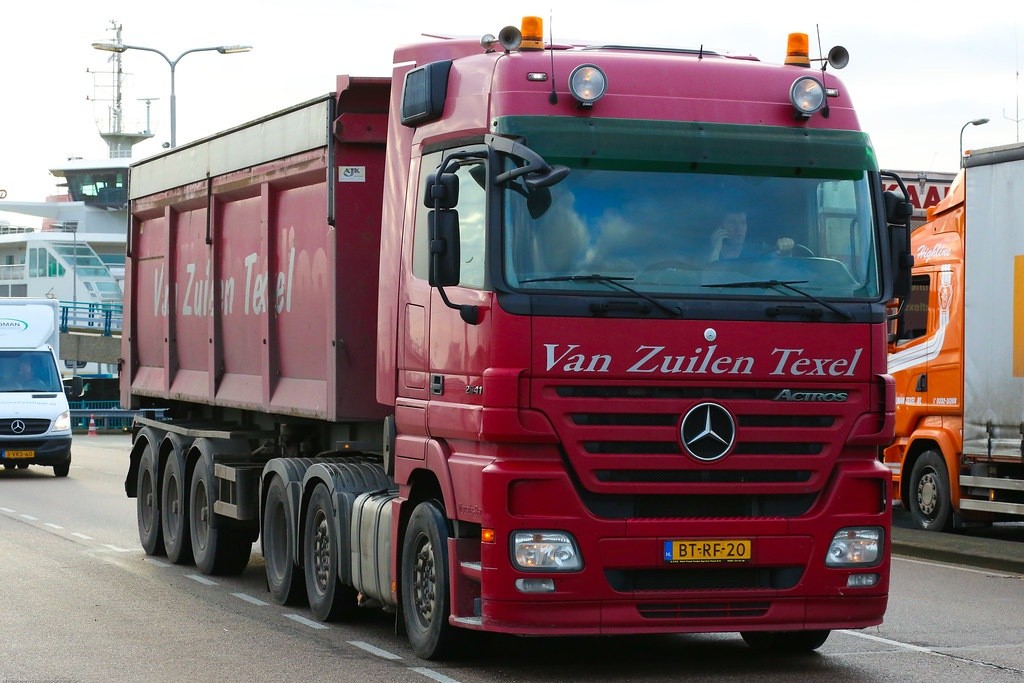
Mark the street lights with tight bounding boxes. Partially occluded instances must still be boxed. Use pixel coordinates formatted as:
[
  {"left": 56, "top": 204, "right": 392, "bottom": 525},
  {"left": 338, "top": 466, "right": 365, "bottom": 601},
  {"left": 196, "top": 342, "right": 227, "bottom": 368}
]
[
  {"left": 93, "top": 42, "right": 253, "bottom": 154},
  {"left": 960, "top": 118, "right": 989, "bottom": 169}
]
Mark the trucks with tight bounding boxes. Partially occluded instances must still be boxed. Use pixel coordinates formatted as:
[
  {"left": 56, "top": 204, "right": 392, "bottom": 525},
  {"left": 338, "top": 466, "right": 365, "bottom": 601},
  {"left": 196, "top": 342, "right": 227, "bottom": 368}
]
[
  {"left": 877, "top": 143, "right": 1024, "bottom": 534},
  {"left": 0, "top": 297, "right": 72, "bottom": 477}
]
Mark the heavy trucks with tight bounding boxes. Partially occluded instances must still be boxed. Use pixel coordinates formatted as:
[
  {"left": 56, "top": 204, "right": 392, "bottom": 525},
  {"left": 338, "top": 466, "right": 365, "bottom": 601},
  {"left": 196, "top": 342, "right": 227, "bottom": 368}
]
[{"left": 118, "top": 27, "right": 913, "bottom": 660}]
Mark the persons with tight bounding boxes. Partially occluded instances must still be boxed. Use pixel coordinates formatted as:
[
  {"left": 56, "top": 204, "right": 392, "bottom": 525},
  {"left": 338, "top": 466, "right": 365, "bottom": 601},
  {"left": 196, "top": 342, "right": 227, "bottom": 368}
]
[
  {"left": 707, "top": 204, "right": 794, "bottom": 263},
  {"left": 7, "top": 358, "right": 49, "bottom": 388}
]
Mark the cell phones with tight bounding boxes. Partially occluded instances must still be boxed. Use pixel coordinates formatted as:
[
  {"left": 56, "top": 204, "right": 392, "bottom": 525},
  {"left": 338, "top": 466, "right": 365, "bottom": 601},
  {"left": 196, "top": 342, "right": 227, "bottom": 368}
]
[{"left": 720, "top": 224, "right": 729, "bottom": 244}]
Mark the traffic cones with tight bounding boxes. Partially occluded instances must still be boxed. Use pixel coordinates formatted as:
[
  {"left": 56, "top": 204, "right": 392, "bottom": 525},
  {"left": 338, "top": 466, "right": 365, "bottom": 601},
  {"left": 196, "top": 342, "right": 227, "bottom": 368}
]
[{"left": 86, "top": 413, "right": 97, "bottom": 437}]
